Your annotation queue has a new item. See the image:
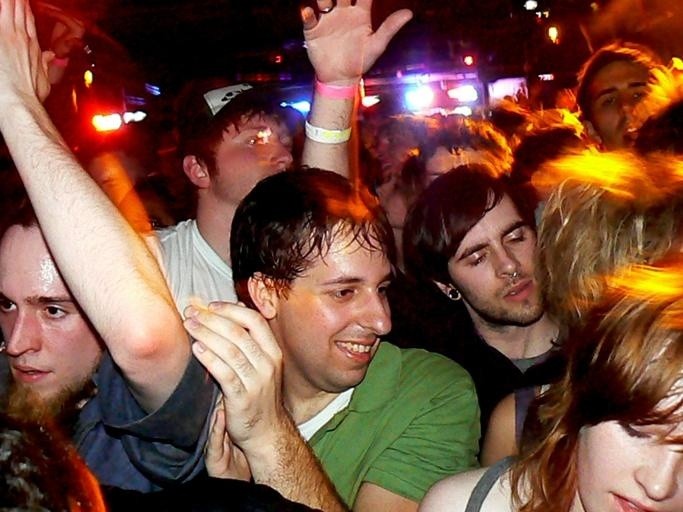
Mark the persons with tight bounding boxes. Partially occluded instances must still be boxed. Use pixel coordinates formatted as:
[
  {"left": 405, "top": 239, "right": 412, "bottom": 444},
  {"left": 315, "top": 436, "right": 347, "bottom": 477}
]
[{"left": 1, "top": 0, "right": 683, "bottom": 510}]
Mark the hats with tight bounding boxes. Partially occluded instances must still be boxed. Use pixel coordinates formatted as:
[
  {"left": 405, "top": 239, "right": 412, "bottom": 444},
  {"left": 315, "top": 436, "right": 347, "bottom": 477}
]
[{"left": 181, "top": 82, "right": 265, "bottom": 138}]
